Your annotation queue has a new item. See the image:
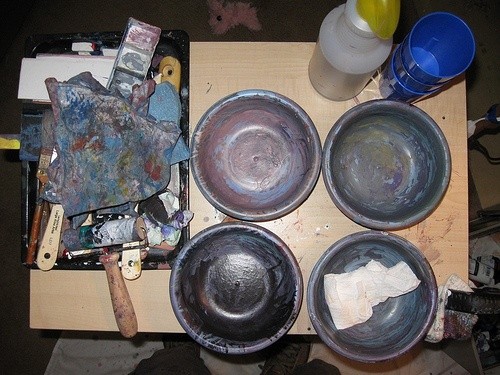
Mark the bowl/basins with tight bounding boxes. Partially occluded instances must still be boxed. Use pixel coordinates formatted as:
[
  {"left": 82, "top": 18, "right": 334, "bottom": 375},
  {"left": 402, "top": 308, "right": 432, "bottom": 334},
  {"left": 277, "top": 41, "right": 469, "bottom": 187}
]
[
  {"left": 306, "top": 230, "right": 438, "bottom": 364},
  {"left": 170, "top": 221, "right": 302, "bottom": 354},
  {"left": 189, "top": 90, "right": 323, "bottom": 221},
  {"left": 322, "top": 99, "right": 451, "bottom": 230}
]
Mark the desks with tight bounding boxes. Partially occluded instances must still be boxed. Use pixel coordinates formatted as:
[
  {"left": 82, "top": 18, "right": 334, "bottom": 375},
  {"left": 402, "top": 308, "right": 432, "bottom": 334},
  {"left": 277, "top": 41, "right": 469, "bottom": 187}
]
[{"left": 30, "top": 41, "right": 469, "bottom": 336}]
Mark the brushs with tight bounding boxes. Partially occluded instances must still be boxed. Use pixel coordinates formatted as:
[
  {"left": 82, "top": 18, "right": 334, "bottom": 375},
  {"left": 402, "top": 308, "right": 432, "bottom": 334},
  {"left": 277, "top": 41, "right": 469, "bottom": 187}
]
[
  {"left": 23, "top": 108, "right": 57, "bottom": 266},
  {"left": 63, "top": 217, "right": 149, "bottom": 339}
]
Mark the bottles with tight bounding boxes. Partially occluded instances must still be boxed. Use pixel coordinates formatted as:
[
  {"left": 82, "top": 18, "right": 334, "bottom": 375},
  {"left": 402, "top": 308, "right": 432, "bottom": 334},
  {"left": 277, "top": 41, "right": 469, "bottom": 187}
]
[{"left": 308, "top": 0, "right": 401, "bottom": 101}]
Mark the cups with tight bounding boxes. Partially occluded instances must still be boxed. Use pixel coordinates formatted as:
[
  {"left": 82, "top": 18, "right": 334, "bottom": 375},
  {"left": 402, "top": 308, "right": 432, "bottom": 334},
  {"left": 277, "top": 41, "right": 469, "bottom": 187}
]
[{"left": 379, "top": 12, "right": 476, "bottom": 104}]
